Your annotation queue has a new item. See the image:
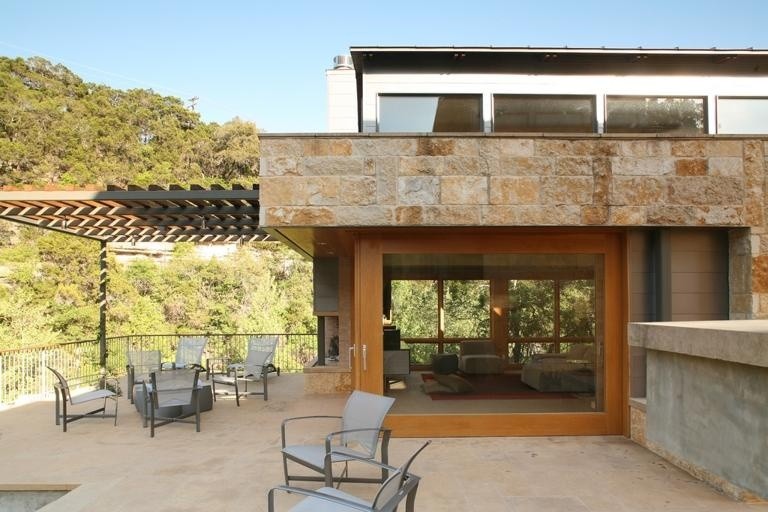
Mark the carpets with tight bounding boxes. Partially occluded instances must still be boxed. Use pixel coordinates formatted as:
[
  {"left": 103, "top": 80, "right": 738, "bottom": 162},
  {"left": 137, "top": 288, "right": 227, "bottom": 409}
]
[{"left": 421, "top": 373, "right": 578, "bottom": 400}]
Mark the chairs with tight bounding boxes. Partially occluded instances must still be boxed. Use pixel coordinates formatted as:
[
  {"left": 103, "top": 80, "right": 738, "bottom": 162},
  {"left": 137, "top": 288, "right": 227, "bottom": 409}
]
[
  {"left": 268, "top": 440, "right": 432, "bottom": 512},
  {"left": 460, "top": 341, "right": 504, "bottom": 375},
  {"left": 282, "top": 389, "right": 396, "bottom": 494},
  {"left": 46, "top": 338, "right": 279, "bottom": 437}
]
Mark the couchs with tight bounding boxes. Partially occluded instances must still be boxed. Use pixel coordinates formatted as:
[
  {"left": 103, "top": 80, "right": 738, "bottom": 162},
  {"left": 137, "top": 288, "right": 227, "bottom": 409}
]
[{"left": 521, "top": 343, "right": 594, "bottom": 393}]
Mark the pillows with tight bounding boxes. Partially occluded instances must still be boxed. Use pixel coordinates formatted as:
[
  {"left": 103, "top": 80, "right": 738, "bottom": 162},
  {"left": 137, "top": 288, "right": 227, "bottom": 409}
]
[
  {"left": 432, "top": 373, "right": 473, "bottom": 392},
  {"left": 424, "top": 381, "right": 455, "bottom": 394}
]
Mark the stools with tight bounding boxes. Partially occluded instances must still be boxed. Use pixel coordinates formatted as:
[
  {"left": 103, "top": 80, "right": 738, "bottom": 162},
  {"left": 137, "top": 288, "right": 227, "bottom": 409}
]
[{"left": 433, "top": 353, "right": 458, "bottom": 374}]
